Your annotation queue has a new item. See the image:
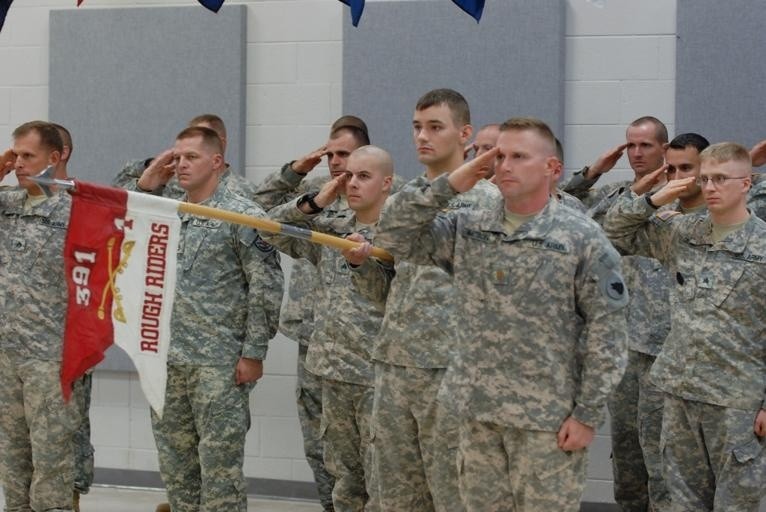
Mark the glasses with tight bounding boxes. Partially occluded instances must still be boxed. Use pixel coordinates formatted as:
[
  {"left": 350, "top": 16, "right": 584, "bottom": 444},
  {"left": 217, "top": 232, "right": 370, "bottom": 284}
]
[{"left": 696, "top": 176, "right": 746, "bottom": 184}]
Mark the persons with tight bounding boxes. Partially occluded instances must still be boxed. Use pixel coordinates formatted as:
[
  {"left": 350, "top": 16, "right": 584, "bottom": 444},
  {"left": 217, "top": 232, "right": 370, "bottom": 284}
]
[
  {"left": 252, "top": 90, "right": 629, "bottom": 512},
  {"left": 52, "top": 124, "right": 94, "bottom": 511},
  {"left": 113, "top": 114, "right": 285, "bottom": 512},
  {"left": 0, "top": 119, "right": 81, "bottom": 511},
  {"left": 557, "top": 116, "right": 765, "bottom": 512}
]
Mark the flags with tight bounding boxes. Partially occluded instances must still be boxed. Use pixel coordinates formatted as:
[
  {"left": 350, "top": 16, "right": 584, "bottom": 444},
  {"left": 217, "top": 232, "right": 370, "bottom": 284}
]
[{"left": 60, "top": 178, "right": 182, "bottom": 421}]
